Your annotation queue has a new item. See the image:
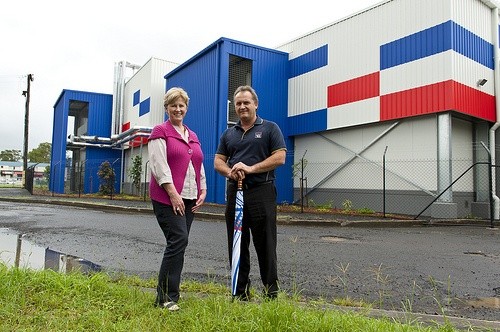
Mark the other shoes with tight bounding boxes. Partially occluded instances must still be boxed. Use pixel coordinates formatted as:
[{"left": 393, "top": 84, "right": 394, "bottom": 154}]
[{"left": 154, "top": 300, "right": 180, "bottom": 310}]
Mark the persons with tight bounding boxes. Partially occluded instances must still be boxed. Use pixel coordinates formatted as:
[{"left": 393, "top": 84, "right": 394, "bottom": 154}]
[
  {"left": 147, "top": 86, "right": 207, "bottom": 313},
  {"left": 214, "top": 86, "right": 288, "bottom": 302}
]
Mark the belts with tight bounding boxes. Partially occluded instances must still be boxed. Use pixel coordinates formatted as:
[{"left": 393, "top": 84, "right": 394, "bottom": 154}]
[{"left": 228, "top": 180, "right": 273, "bottom": 190}]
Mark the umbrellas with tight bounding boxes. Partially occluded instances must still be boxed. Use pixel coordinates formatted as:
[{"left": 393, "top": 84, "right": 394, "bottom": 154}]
[{"left": 230, "top": 174, "right": 244, "bottom": 304}]
